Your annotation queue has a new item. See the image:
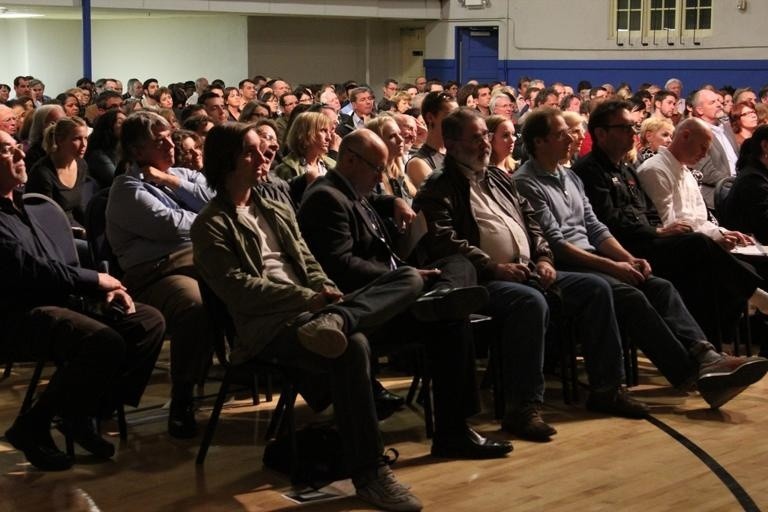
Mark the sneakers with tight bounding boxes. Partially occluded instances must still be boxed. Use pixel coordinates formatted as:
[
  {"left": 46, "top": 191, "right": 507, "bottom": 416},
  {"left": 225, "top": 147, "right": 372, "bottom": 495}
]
[
  {"left": 354, "top": 459, "right": 424, "bottom": 512},
  {"left": 695, "top": 350, "right": 768, "bottom": 412},
  {"left": 296, "top": 314, "right": 350, "bottom": 361},
  {"left": 4, "top": 411, "right": 74, "bottom": 474},
  {"left": 54, "top": 416, "right": 115, "bottom": 460},
  {"left": 169, "top": 398, "right": 201, "bottom": 441}
]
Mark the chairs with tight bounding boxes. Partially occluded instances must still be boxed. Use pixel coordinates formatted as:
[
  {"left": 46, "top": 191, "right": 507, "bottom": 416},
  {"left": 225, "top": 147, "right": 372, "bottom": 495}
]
[
  {"left": 1, "top": 191, "right": 129, "bottom": 461},
  {"left": 86, "top": 186, "right": 752, "bottom": 466}
]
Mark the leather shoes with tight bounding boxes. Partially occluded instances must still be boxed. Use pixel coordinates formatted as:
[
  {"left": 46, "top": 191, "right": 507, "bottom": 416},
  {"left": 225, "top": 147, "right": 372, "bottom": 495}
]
[
  {"left": 585, "top": 386, "right": 652, "bottom": 416},
  {"left": 500, "top": 402, "right": 558, "bottom": 440},
  {"left": 430, "top": 422, "right": 515, "bottom": 461},
  {"left": 369, "top": 376, "right": 407, "bottom": 423}
]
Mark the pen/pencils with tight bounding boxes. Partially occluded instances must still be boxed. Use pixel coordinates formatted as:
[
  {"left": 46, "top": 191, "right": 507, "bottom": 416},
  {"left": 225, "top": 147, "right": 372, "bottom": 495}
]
[{"left": 719, "top": 229, "right": 738, "bottom": 250}]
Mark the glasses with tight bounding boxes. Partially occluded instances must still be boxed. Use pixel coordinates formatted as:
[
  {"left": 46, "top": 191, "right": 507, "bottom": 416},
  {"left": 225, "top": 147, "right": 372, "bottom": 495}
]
[
  {"left": 347, "top": 148, "right": 386, "bottom": 177},
  {"left": 251, "top": 111, "right": 271, "bottom": 121},
  {"left": 0, "top": 143, "right": 24, "bottom": 159},
  {"left": 740, "top": 111, "right": 757, "bottom": 117},
  {"left": 451, "top": 132, "right": 494, "bottom": 146},
  {"left": 495, "top": 103, "right": 514, "bottom": 108},
  {"left": 600, "top": 121, "right": 635, "bottom": 130},
  {"left": 283, "top": 101, "right": 300, "bottom": 106},
  {"left": 299, "top": 98, "right": 312, "bottom": 102},
  {"left": 103, "top": 100, "right": 128, "bottom": 110}
]
